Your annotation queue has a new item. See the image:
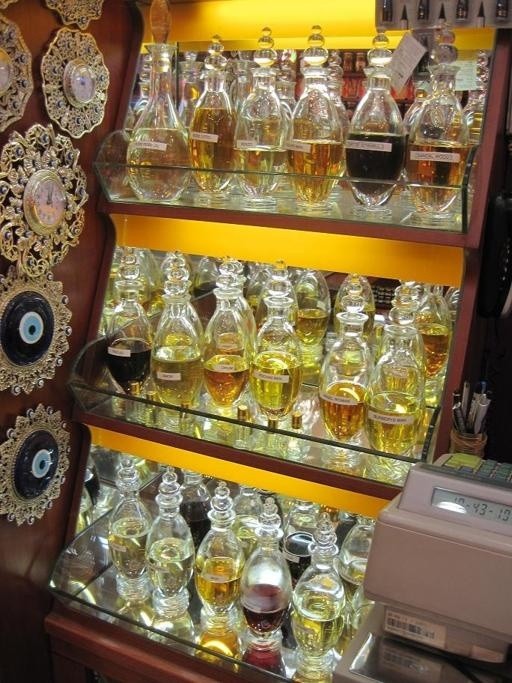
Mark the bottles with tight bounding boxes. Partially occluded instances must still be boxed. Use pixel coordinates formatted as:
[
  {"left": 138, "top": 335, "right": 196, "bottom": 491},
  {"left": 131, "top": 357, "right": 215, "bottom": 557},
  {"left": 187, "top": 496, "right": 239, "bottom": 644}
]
[
  {"left": 63, "top": 442, "right": 152, "bottom": 617},
  {"left": 126, "top": 0, "right": 491, "bottom": 224},
  {"left": 101, "top": 452, "right": 375, "bottom": 683},
  {"left": 316, "top": 458, "right": 409, "bottom": 484},
  {"left": 99, "top": 245, "right": 462, "bottom": 457}
]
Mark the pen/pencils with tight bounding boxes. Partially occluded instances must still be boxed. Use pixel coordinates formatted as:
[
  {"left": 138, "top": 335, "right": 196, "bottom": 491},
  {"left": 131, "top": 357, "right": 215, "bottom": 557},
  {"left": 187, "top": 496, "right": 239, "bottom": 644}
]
[{"left": 451, "top": 380, "right": 492, "bottom": 435}]
